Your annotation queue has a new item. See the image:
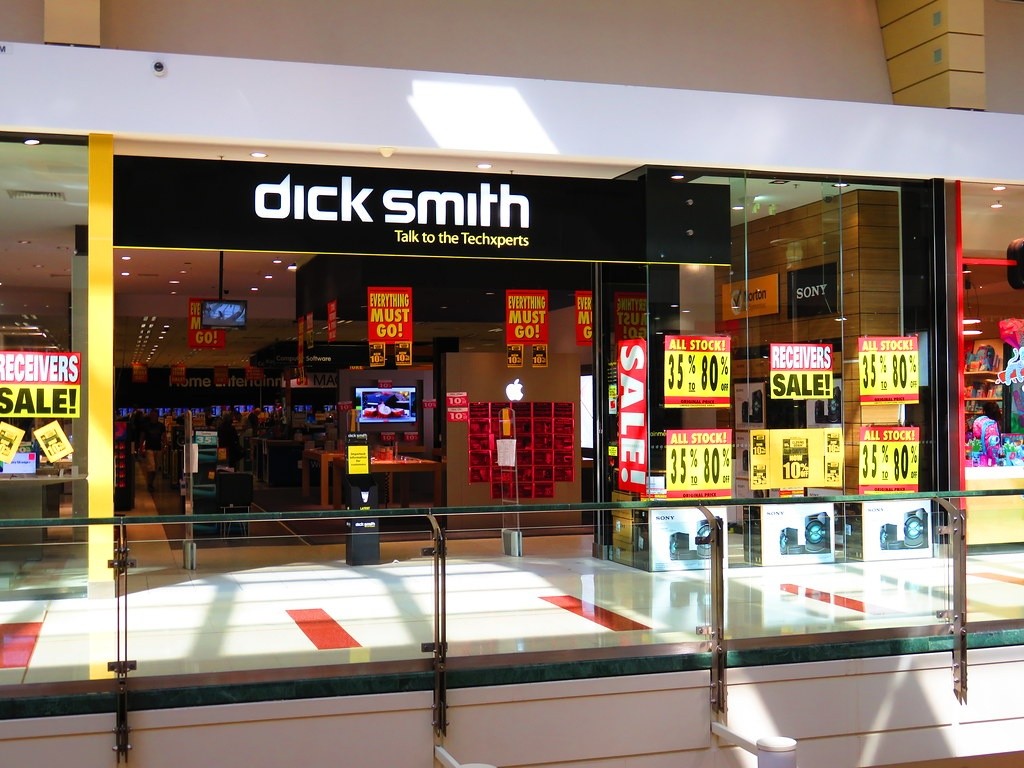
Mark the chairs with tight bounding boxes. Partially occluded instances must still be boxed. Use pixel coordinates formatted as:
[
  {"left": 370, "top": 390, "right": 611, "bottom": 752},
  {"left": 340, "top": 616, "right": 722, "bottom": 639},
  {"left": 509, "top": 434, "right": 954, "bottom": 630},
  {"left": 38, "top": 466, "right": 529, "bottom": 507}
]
[{"left": 217, "top": 472, "right": 254, "bottom": 542}]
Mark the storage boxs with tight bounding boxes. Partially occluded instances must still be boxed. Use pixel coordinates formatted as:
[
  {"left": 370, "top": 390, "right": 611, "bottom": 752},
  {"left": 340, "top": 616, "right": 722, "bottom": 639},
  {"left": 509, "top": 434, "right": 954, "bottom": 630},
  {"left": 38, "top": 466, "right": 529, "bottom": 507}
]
[
  {"left": 834, "top": 498, "right": 935, "bottom": 562},
  {"left": 611, "top": 491, "right": 636, "bottom": 567},
  {"left": 632, "top": 506, "right": 729, "bottom": 572},
  {"left": 741, "top": 503, "right": 835, "bottom": 567}
]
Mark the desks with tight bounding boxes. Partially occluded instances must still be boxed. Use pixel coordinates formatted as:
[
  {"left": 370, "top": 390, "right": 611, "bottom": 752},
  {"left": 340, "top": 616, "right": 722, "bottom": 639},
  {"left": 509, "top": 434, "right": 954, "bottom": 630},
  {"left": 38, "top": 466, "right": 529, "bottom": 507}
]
[
  {"left": 302, "top": 448, "right": 346, "bottom": 508},
  {"left": 0, "top": 473, "right": 88, "bottom": 560},
  {"left": 332, "top": 456, "right": 443, "bottom": 521}
]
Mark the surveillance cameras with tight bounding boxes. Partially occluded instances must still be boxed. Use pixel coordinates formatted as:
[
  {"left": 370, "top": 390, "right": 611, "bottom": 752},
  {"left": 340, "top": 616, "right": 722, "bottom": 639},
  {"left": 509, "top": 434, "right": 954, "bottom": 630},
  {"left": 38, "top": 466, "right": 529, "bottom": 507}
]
[{"left": 823, "top": 195, "right": 833, "bottom": 203}]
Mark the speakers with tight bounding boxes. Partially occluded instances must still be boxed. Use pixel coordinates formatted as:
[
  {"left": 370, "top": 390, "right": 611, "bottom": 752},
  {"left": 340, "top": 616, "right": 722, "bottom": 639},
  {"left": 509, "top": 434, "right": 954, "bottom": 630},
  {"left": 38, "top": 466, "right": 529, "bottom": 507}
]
[
  {"left": 879, "top": 508, "right": 928, "bottom": 550},
  {"left": 780, "top": 511, "right": 831, "bottom": 554},
  {"left": 670, "top": 516, "right": 723, "bottom": 560}
]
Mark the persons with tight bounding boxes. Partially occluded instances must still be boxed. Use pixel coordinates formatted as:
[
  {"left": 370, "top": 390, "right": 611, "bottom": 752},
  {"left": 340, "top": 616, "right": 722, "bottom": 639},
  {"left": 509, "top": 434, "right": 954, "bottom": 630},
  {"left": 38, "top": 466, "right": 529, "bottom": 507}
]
[
  {"left": 162, "top": 412, "right": 167, "bottom": 416},
  {"left": 217, "top": 415, "right": 238, "bottom": 448},
  {"left": 230, "top": 405, "right": 242, "bottom": 418},
  {"left": 248, "top": 408, "right": 261, "bottom": 437},
  {"left": 139, "top": 410, "right": 167, "bottom": 493},
  {"left": 133, "top": 410, "right": 144, "bottom": 458},
  {"left": 980, "top": 399, "right": 1003, "bottom": 437},
  {"left": 176, "top": 412, "right": 184, "bottom": 425},
  {"left": 276, "top": 401, "right": 282, "bottom": 417}
]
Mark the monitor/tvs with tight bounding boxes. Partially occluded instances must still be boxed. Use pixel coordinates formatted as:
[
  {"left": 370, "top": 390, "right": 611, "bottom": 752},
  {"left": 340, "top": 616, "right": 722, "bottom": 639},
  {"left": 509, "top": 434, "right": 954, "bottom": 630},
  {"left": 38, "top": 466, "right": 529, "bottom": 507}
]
[
  {"left": 787, "top": 261, "right": 839, "bottom": 318},
  {"left": 354, "top": 386, "right": 417, "bottom": 424},
  {"left": 201, "top": 299, "right": 247, "bottom": 327}
]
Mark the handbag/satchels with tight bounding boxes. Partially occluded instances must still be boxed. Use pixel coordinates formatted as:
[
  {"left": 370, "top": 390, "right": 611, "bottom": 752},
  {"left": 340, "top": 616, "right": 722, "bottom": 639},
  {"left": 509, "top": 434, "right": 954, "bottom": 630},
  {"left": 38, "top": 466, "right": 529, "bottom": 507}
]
[{"left": 234, "top": 440, "right": 245, "bottom": 461}]
